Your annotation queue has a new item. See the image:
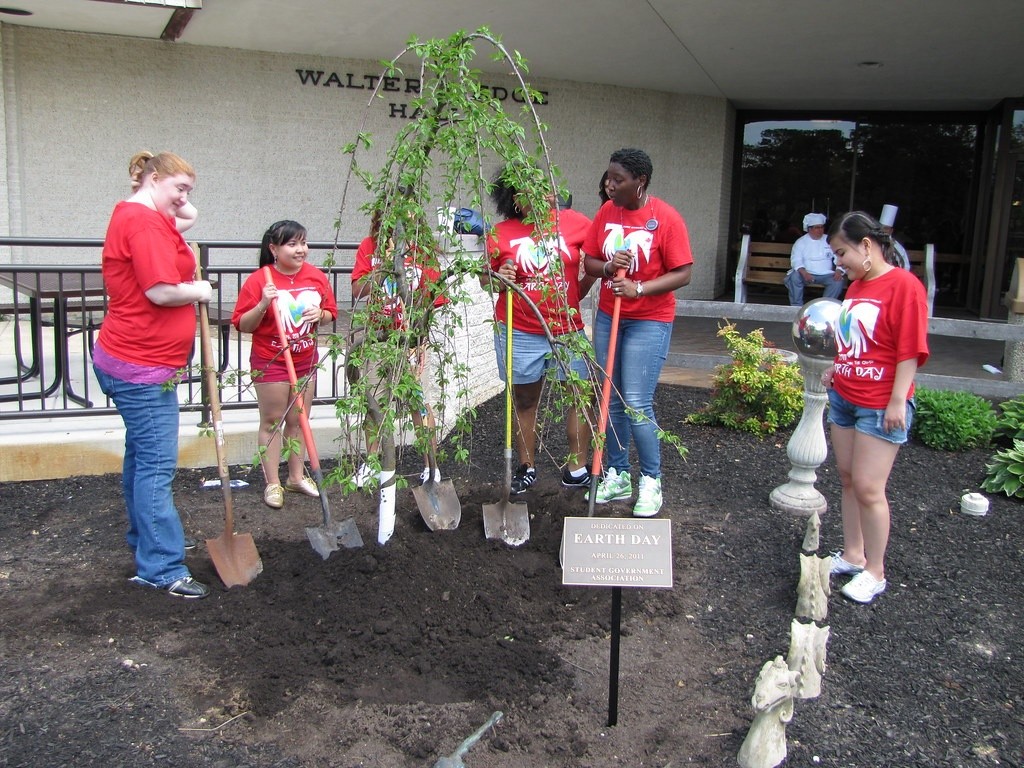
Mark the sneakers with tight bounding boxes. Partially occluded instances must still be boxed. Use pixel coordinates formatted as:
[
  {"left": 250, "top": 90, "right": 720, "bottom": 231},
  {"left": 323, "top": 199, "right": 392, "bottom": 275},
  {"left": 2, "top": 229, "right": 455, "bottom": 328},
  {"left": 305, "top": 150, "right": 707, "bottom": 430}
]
[
  {"left": 350, "top": 462, "right": 380, "bottom": 488},
  {"left": 584, "top": 466, "right": 634, "bottom": 504},
  {"left": 421, "top": 467, "right": 440, "bottom": 484},
  {"left": 264, "top": 484, "right": 284, "bottom": 508},
  {"left": 842, "top": 568, "right": 886, "bottom": 603},
  {"left": 510, "top": 462, "right": 539, "bottom": 495},
  {"left": 831, "top": 552, "right": 864, "bottom": 575},
  {"left": 561, "top": 465, "right": 593, "bottom": 487},
  {"left": 285, "top": 475, "right": 321, "bottom": 497},
  {"left": 633, "top": 471, "right": 663, "bottom": 515},
  {"left": 156, "top": 574, "right": 209, "bottom": 598}
]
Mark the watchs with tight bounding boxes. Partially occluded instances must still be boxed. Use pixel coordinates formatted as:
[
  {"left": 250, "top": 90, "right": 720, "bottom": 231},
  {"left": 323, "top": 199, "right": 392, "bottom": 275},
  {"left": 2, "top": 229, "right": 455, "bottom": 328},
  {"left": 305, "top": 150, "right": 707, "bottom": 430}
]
[{"left": 636, "top": 282, "right": 642, "bottom": 298}]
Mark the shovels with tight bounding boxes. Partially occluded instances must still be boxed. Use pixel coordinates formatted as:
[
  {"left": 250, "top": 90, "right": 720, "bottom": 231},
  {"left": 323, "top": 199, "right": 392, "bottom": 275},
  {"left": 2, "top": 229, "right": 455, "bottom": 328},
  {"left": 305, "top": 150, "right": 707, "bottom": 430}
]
[
  {"left": 263, "top": 266, "right": 365, "bottom": 559},
  {"left": 190, "top": 241, "right": 263, "bottom": 589},
  {"left": 559, "top": 265, "right": 627, "bottom": 572},
  {"left": 482, "top": 258, "right": 530, "bottom": 547},
  {"left": 401, "top": 297, "right": 461, "bottom": 532}
]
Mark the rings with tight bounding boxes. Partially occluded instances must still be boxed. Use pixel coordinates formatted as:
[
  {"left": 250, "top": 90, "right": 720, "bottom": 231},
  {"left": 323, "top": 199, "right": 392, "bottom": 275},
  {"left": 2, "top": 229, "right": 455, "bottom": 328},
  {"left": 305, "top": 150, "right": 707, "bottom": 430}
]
[
  {"left": 825, "top": 373, "right": 828, "bottom": 376},
  {"left": 614, "top": 288, "right": 619, "bottom": 292}
]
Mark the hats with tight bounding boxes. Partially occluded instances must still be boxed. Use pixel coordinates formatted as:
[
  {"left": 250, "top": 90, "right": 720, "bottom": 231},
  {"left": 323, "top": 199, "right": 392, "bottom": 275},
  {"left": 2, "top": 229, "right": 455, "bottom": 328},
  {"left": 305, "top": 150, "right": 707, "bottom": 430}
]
[
  {"left": 803, "top": 213, "right": 827, "bottom": 232},
  {"left": 879, "top": 205, "right": 898, "bottom": 228}
]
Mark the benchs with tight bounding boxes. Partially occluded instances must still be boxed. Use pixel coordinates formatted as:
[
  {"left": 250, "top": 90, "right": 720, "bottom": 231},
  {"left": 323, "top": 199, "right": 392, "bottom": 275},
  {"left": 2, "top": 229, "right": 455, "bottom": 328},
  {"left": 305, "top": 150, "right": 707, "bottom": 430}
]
[
  {"left": 0, "top": 296, "right": 242, "bottom": 402},
  {"left": 734, "top": 234, "right": 937, "bottom": 318}
]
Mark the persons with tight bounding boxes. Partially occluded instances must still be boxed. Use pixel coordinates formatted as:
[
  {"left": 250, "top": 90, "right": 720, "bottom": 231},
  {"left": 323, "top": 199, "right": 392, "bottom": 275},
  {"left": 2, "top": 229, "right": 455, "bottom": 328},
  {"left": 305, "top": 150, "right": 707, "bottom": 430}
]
[
  {"left": 350, "top": 187, "right": 450, "bottom": 489},
  {"left": 232, "top": 220, "right": 337, "bottom": 508},
  {"left": 799, "top": 314, "right": 835, "bottom": 349},
  {"left": 598, "top": 168, "right": 610, "bottom": 207},
  {"left": 784, "top": 213, "right": 846, "bottom": 307},
  {"left": 478, "top": 160, "right": 601, "bottom": 494},
  {"left": 584, "top": 147, "right": 693, "bottom": 516},
  {"left": 879, "top": 204, "right": 911, "bottom": 271},
  {"left": 92, "top": 150, "right": 213, "bottom": 599},
  {"left": 820, "top": 211, "right": 930, "bottom": 603}
]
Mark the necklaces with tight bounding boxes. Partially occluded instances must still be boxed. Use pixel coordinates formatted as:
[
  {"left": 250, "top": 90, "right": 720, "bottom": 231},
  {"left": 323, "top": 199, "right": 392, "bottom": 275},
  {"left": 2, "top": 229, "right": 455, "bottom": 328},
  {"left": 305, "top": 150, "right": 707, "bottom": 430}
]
[{"left": 275, "top": 265, "right": 302, "bottom": 284}]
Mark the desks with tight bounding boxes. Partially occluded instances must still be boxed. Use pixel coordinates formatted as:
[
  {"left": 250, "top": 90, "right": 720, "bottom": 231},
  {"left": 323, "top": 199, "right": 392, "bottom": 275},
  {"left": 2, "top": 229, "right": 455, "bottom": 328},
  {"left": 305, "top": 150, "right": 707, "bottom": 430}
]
[{"left": 0, "top": 268, "right": 111, "bottom": 408}]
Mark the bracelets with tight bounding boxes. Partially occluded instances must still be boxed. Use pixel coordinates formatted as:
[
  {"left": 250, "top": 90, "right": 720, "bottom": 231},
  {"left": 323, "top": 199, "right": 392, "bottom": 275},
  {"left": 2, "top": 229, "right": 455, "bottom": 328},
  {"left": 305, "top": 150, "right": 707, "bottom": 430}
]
[
  {"left": 603, "top": 261, "right": 611, "bottom": 279},
  {"left": 258, "top": 304, "right": 267, "bottom": 314},
  {"left": 321, "top": 310, "right": 326, "bottom": 319}
]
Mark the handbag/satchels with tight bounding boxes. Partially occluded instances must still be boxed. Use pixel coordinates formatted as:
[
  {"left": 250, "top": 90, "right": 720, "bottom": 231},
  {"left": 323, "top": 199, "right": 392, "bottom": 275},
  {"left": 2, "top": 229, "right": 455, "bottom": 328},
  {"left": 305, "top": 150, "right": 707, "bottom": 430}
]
[{"left": 453, "top": 208, "right": 489, "bottom": 238}]
